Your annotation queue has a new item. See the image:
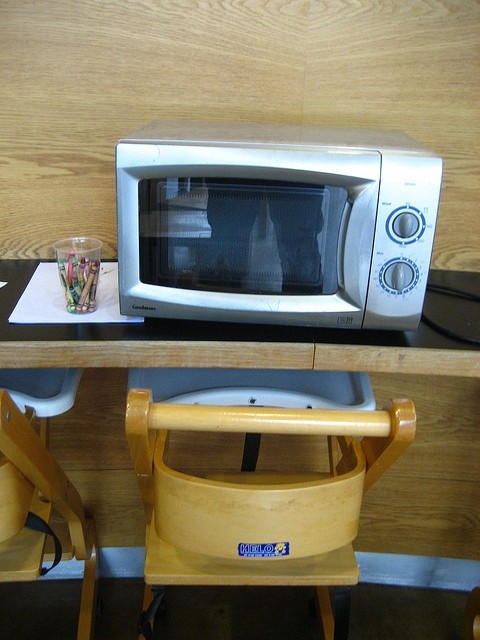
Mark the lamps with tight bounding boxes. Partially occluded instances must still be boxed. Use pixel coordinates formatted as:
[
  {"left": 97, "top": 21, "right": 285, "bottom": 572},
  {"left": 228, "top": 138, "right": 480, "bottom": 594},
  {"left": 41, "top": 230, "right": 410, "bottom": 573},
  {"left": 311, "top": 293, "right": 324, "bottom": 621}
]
[
  {"left": 126, "top": 369, "right": 416, "bottom": 640},
  {"left": 0, "top": 367, "right": 98, "bottom": 640}
]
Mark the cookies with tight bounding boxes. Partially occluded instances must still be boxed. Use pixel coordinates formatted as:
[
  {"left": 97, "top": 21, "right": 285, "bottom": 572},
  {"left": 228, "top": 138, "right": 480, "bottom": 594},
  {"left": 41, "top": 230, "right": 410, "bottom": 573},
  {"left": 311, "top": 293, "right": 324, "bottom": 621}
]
[{"left": 115, "top": 120, "right": 445, "bottom": 331}]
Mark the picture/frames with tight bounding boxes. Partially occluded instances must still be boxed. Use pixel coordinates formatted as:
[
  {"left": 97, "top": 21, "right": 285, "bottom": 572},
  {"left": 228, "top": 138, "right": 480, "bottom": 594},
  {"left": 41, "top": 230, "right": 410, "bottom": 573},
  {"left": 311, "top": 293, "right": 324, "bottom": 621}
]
[{"left": 1, "top": 260, "right": 480, "bottom": 640}]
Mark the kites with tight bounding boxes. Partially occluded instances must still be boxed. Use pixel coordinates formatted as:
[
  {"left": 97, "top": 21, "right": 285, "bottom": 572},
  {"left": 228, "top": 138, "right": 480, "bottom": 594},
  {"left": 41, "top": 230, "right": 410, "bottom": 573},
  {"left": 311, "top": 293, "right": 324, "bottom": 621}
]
[{"left": 58, "top": 248, "right": 100, "bottom": 314}]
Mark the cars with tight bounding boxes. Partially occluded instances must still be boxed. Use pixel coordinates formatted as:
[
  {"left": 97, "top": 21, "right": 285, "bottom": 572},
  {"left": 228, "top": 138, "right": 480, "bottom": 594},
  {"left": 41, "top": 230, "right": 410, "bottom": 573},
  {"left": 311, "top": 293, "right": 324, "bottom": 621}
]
[{"left": 53, "top": 236, "right": 103, "bottom": 314}]
